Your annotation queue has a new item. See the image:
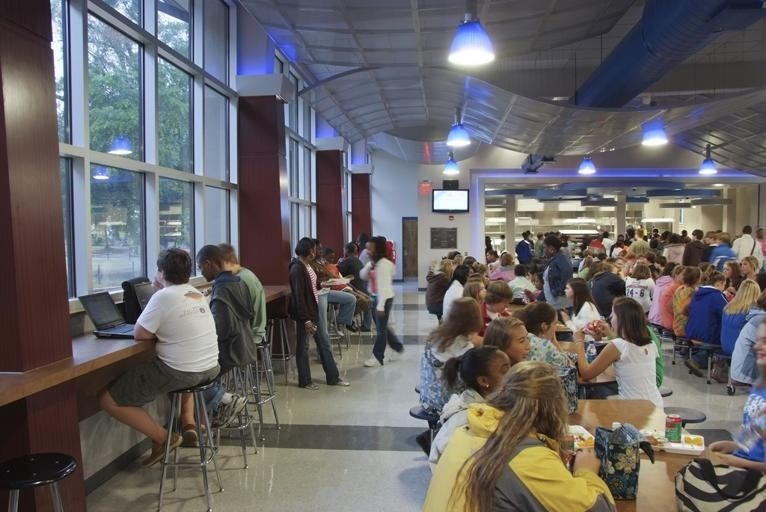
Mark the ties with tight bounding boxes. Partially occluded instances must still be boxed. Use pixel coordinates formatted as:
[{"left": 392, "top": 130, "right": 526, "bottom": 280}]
[{"left": 432, "top": 189, "right": 469, "bottom": 213}]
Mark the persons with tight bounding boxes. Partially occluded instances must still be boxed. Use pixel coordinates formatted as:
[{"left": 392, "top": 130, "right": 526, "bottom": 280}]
[
  {"left": 357, "top": 235, "right": 403, "bottom": 367},
  {"left": 310, "top": 234, "right": 373, "bottom": 336},
  {"left": 417, "top": 222, "right": 765, "bottom": 511},
  {"left": 195, "top": 244, "right": 256, "bottom": 432},
  {"left": 287, "top": 237, "right": 351, "bottom": 391},
  {"left": 96, "top": 247, "right": 221, "bottom": 469},
  {"left": 216, "top": 243, "right": 267, "bottom": 344}
]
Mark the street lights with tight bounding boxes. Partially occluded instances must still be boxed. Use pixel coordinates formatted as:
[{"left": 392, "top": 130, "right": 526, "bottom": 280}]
[
  {"left": 594, "top": 423, "right": 655, "bottom": 502},
  {"left": 672, "top": 457, "right": 766, "bottom": 512}
]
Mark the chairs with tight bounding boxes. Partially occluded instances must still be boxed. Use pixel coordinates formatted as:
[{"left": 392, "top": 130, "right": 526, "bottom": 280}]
[
  {"left": 297, "top": 381, "right": 319, "bottom": 391},
  {"left": 684, "top": 358, "right": 704, "bottom": 378},
  {"left": 389, "top": 347, "right": 407, "bottom": 362},
  {"left": 210, "top": 392, "right": 249, "bottom": 430},
  {"left": 363, "top": 352, "right": 384, "bottom": 367},
  {"left": 179, "top": 423, "right": 198, "bottom": 448},
  {"left": 327, "top": 376, "right": 350, "bottom": 386},
  {"left": 140, "top": 432, "right": 183, "bottom": 470}
]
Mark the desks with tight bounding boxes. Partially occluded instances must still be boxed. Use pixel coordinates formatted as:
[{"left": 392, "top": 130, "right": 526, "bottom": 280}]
[{"left": 507, "top": 301, "right": 712, "bottom": 512}]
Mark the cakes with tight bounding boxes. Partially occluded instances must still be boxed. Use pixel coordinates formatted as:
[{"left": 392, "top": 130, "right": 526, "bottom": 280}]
[
  {"left": 587, "top": 354, "right": 595, "bottom": 363},
  {"left": 665, "top": 414, "right": 683, "bottom": 443},
  {"left": 562, "top": 435, "right": 574, "bottom": 469}
]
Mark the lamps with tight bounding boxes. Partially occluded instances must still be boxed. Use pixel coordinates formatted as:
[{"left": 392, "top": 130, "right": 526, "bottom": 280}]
[
  {"left": 446, "top": 108, "right": 471, "bottom": 147},
  {"left": 446, "top": 1, "right": 496, "bottom": 70},
  {"left": 698, "top": 144, "right": 717, "bottom": 175},
  {"left": 577, "top": 154, "right": 596, "bottom": 174},
  {"left": 641, "top": 128, "right": 668, "bottom": 146},
  {"left": 441, "top": 151, "right": 460, "bottom": 175}
]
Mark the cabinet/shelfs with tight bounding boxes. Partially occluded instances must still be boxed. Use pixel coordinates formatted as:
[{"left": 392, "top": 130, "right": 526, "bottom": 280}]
[
  {"left": 587, "top": 340, "right": 597, "bottom": 363},
  {"left": 612, "top": 422, "right": 622, "bottom": 431},
  {"left": 309, "top": 325, "right": 317, "bottom": 337}
]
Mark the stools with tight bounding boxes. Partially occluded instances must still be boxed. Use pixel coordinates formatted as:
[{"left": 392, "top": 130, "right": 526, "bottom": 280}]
[
  {"left": 408, "top": 311, "right": 443, "bottom": 452},
  {"left": 646, "top": 312, "right": 736, "bottom": 448},
  {"left": 0, "top": 256, "right": 374, "bottom": 512}
]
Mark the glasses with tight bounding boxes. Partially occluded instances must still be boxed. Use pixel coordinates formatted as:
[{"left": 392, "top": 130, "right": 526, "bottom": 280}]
[
  {"left": 446, "top": 108, "right": 471, "bottom": 147},
  {"left": 577, "top": 154, "right": 596, "bottom": 174},
  {"left": 446, "top": 1, "right": 496, "bottom": 70},
  {"left": 698, "top": 144, "right": 717, "bottom": 175},
  {"left": 441, "top": 151, "right": 460, "bottom": 175},
  {"left": 641, "top": 128, "right": 668, "bottom": 146}
]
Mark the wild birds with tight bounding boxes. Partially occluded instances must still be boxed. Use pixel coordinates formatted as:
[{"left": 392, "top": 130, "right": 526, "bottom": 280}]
[
  {"left": 79, "top": 291, "right": 136, "bottom": 339},
  {"left": 134, "top": 281, "right": 156, "bottom": 312}
]
